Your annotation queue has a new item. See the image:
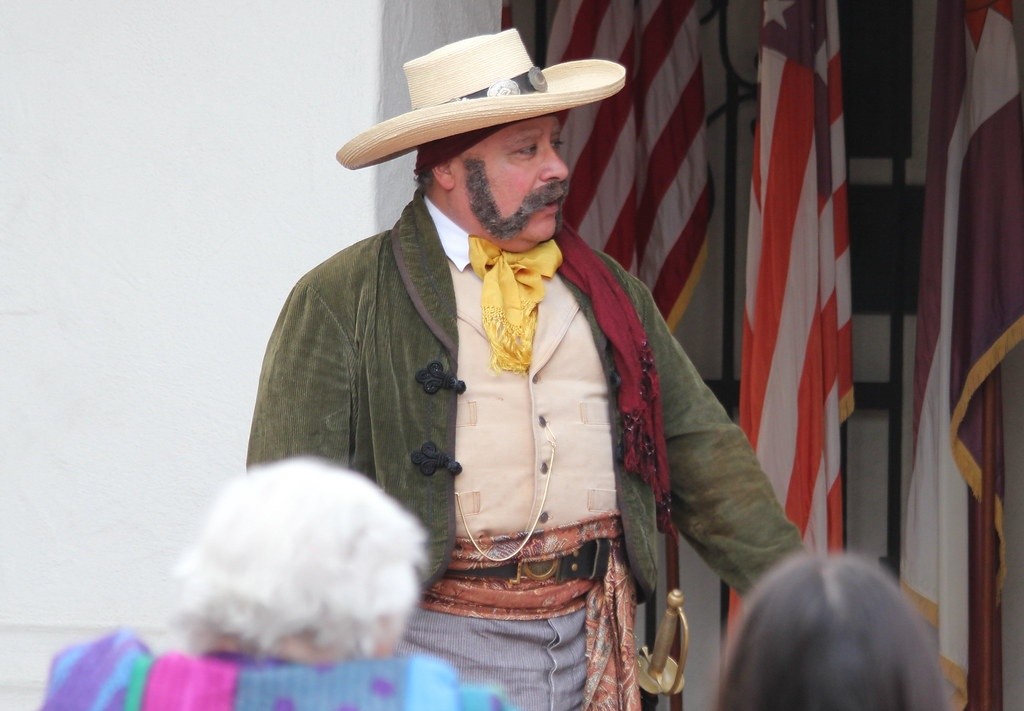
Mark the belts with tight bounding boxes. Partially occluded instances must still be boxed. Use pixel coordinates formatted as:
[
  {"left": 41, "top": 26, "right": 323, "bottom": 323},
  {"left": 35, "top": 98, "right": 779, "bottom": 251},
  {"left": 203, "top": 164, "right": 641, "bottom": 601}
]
[{"left": 443, "top": 537, "right": 613, "bottom": 581}]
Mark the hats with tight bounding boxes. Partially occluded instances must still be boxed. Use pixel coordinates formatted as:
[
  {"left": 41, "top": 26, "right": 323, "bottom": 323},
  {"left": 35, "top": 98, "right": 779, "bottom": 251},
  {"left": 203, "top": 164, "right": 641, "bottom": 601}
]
[{"left": 336, "top": 27, "right": 629, "bottom": 170}]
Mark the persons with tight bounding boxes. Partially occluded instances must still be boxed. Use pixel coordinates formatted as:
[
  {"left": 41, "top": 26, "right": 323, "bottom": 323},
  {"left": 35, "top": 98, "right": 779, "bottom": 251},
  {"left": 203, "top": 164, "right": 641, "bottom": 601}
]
[
  {"left": 36, "top": 456, "right": 517, "bottom": 711},
  {"left": 713, "top": 555, "right": 949, "bottom": 711},
  {"left": 245, "top": 29, "right": 803, "bottom": 711}
]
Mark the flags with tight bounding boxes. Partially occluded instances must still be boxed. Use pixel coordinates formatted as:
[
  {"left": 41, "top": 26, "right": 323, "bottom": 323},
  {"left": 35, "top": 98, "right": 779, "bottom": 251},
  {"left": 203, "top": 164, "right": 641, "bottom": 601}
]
[
  {"left": 902, "top": 0, "right": 1024, "bottom": 711},
  {"left": 546, "top": 0, "right": 714, "bottom": 330},
  {"left": 728, "top": 0, "right": 856, "bottom": 644}
]
